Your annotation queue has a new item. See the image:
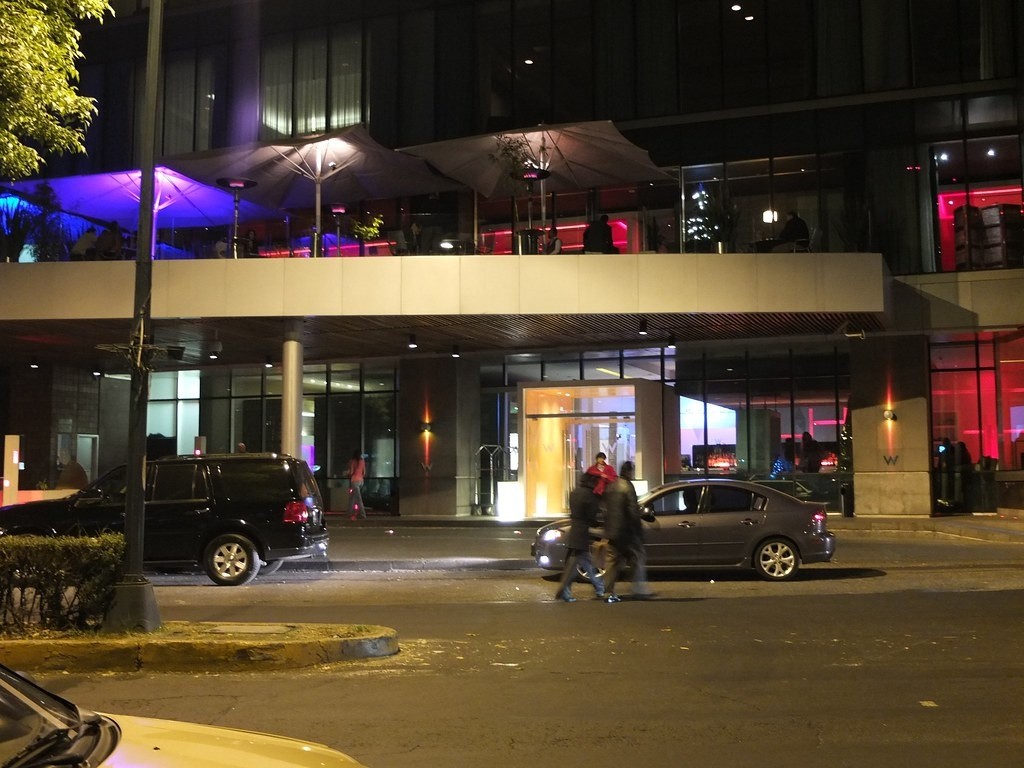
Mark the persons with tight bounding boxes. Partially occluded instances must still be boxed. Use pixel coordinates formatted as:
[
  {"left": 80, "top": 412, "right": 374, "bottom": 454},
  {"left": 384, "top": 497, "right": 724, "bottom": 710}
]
[
  {"left": 556, "top": 471, "right": 604, "bottom": 602},
  {"left": 677, "top": 490, "right": 698, "bottom": 514},
  {"left": 585, "top": 452, "right": 618, "bottom": 496},
  {"left": 937, "top": 437, "right": 976, "bottom": 508},
  {"left": 70, "top": 221, "right": 125, "bottom": 262},
  {"left": 546, "top": 226, "right": 562, "bottom": 255},
  {"left": 772, "top": 211, "right": 809, "bottom": 253},
  {"left": 785, "top": 431, "right": 822, "bottom": 473},
  {"left": 244, "top": 228, "right": 259, "bottom": 258},
  {"left": 602, "top": 461, "right": 661, "bottom": 604},
  {"left": 344, "top": 449, "right": 367, "bottom": 518},
  {"left": 582, "top": 215, "right": 620, "bottom": 254}
]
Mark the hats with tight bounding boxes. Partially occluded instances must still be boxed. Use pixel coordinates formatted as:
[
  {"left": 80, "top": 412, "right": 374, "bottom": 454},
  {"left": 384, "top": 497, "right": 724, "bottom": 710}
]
[{"left": 595, "top": 452, "right": 606, "bottom": 459}]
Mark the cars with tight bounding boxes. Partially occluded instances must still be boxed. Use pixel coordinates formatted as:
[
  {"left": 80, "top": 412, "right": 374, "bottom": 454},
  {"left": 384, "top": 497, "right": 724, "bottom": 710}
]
[
  {"left": 532, "top": 479, "right": 840, "bottom": 583},
  {"left": 747, "top": 473, "right": 846, "bottom": 511},
  {"left": 1, "top": 658, "right": 371, "bottom": 768}
]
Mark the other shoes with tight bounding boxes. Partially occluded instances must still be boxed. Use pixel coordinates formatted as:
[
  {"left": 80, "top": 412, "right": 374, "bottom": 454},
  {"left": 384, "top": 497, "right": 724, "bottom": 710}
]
[
  {"left": 555, "top": 592, "right": 577, "bottom": 602},
  {"left": 604, "top": 594, "right": 623, "bottom": 602},
  {"left": 635, "top": 594, "right": 653, "bottom": 601},
  {"left": 595, "top": 589, "right": 607, "bottom": 597}
]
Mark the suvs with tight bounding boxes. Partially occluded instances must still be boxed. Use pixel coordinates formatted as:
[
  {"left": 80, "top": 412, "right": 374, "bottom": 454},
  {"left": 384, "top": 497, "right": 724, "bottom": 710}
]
[{"left": 1, "top": 453, "right": 330, "bottom": 587}]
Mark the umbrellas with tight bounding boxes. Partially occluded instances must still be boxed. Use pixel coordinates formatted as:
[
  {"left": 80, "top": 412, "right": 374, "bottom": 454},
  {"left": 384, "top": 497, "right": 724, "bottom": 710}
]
[
  {"left": 0, "top": 166, "right": 288, "bottom": 260},
  {"left": 158, "top": 120, "right": 470, "bottom": 259},
  {"left": 395, "top": 119, "right": 680, "bottom": 254}
]
